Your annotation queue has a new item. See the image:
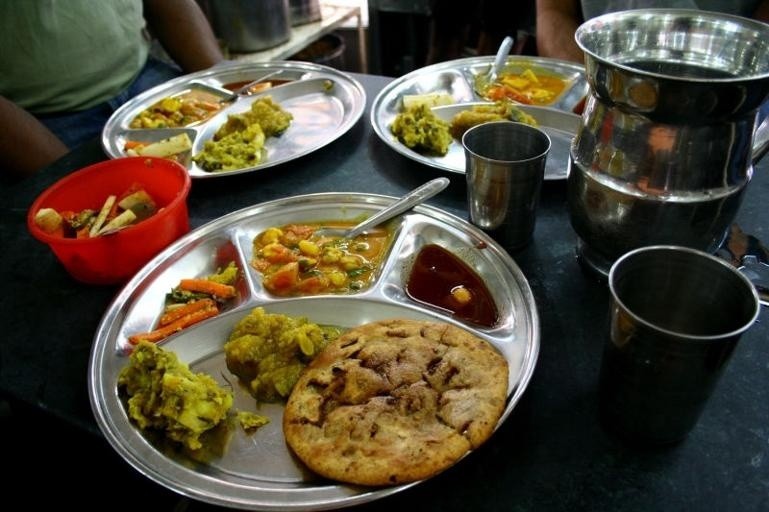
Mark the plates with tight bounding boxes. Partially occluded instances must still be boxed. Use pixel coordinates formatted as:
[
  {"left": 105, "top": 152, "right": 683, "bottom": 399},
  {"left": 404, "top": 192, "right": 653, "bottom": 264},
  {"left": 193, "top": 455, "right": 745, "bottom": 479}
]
[
  {"left": 88, "top": 192, "right": 542, "bottom": 510},
  {"left": 371, "top": 54, "right": 595, "bottom": 181},
  {"left": 102, "top": 60, "right": 367, "bottom": 178}
]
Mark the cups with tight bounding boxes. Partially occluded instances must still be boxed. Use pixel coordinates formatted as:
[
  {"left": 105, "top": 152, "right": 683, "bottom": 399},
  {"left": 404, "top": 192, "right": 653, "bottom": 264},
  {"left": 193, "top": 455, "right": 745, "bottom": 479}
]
[
  {"left": 461, "top": 121, "right": 553, "bottom": 239},
  {"left": 591, "top": 244, "right": 759, "bottom": 455}
]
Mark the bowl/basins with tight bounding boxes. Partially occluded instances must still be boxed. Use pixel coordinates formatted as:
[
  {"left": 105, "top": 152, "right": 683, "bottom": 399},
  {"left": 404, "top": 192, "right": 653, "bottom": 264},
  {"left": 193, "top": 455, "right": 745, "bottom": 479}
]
[{"left": 28, "top": 156, "right": 192, "bottom": 286}]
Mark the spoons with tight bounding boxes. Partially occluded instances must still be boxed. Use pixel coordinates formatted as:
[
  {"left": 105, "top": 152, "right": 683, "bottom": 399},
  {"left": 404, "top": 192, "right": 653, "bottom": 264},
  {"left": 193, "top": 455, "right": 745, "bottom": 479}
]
[
  {"left": 473, "top": 37, "right": 516, "bottom": 98},
  {"left": 311, "top": 178, "right": 451, "bottom": 247}
]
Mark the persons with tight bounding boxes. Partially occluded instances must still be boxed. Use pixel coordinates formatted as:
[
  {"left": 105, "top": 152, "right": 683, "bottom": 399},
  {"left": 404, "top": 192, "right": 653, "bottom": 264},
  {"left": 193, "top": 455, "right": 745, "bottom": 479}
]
[
  {"left": 535, "top": 0, "right": 769, "bottom": 64},
  {"left": 1, "top": 1, "right": 221, "bottom": 177}
]
